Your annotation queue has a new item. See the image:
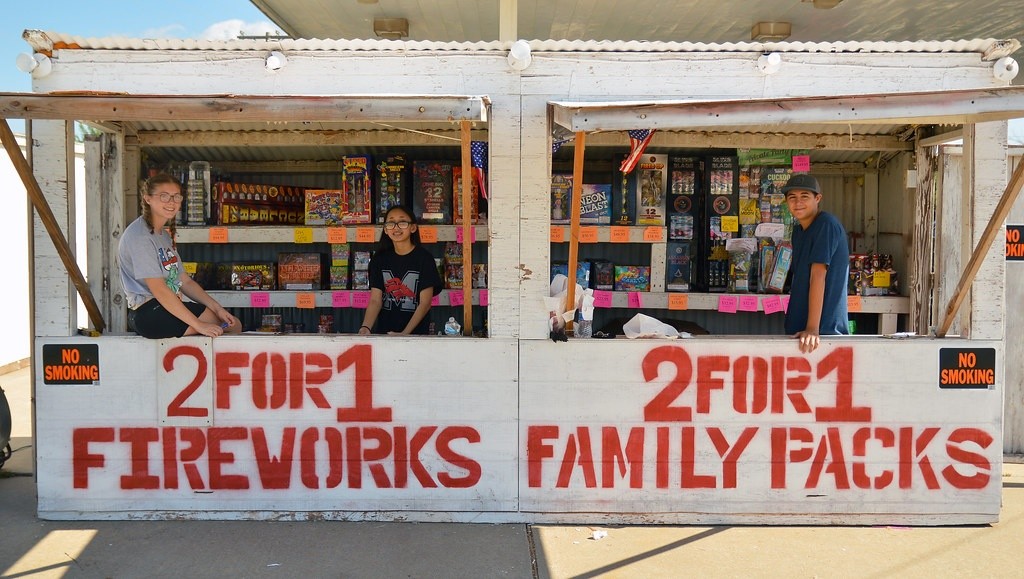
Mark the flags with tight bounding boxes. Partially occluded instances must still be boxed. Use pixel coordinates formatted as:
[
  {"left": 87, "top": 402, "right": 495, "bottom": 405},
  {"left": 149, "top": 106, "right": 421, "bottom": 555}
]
[
  {"left": 619, "top": 129, "right": 656, "bottom": 174},
  {"left": 471, "top": 140, "right": 489, "bottom": 198}
]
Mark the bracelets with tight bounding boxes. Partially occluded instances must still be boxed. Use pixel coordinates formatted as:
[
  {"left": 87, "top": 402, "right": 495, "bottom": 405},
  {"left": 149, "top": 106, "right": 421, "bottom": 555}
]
[{"left": 360, "top": 326, "right": 372, "bottom": 334}]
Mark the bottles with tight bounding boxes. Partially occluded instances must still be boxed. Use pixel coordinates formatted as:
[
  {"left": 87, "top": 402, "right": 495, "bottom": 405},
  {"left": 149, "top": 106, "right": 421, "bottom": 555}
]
[
  {"left": 710, "top": 217, "right": 732, "bottom": 286},
  {"left": 670, "top": 216, "right": 693, "bottom": 239},
  {"left": 445, "top": 317, "right": 460, "bottom": 336}
]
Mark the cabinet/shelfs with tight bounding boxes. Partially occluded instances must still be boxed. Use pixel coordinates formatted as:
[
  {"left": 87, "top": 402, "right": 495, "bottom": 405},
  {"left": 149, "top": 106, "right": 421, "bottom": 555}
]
[{"left": 174, "top": 225, "right": 666, "bottom": 306}]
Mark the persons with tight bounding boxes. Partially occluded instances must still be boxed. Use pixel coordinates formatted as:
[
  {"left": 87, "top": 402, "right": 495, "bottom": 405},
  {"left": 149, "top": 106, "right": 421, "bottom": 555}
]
[
  {"left": 118, "top": 175, "right": 241, "bottom": 340},
  {"left": 358, "top": 205, "right": 442, "bottom": 335},
  {"left": 781, "top": 174, "right": 849, "bottom": 354}
]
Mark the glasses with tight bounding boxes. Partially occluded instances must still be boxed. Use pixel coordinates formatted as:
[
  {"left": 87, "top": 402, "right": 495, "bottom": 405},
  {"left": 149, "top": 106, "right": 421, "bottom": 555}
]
[
  {"left": 383, "top": 221, "right": 413, "bottom": 230},
  {"left": 151, "top": 192, "right": 184, "bottom": 203}
]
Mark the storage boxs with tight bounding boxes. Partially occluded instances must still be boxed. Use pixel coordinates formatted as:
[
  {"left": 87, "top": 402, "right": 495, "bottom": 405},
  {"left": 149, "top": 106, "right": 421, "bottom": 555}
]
[{"left": 139, "top": 149, "right": 810, "bottom": 291}]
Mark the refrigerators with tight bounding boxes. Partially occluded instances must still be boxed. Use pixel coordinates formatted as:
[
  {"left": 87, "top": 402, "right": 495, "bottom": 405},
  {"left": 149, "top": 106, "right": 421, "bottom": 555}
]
[{"left": 667, "top": 148, "right": 742, "bottom": 293}]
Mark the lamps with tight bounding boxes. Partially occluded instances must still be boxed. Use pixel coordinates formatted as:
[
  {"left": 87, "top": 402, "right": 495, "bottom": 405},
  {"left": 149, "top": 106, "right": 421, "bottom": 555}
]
[
  {"left": 759, "top": 51, "right": 783, "bottom": 73},
  {"left": 15, "top": 52, "right": 52, "bottom": 78},
  {"left": 992, "top": 56, "right": 1019, "bottom": 80},
  {"left": 264, "top": 50, "right": 286, "bottom": 71},
  {"left": 507, "top": 42, "right": 532, "bottom": 69}
]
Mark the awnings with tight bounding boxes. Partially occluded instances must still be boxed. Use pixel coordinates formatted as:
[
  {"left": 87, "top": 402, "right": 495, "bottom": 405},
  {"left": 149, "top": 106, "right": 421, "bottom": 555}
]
[
  {"left": 547, "top": 85, "right": 1024, "bottom": 134},
  {"left": 0, "top": 95, "right": 487, "bottom": 132}
]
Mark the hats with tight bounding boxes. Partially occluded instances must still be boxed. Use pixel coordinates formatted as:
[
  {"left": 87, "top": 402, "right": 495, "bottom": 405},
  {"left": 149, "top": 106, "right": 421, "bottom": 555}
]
[{"left": 781, "top": 174, "right": 820, "bottom": 195}]
